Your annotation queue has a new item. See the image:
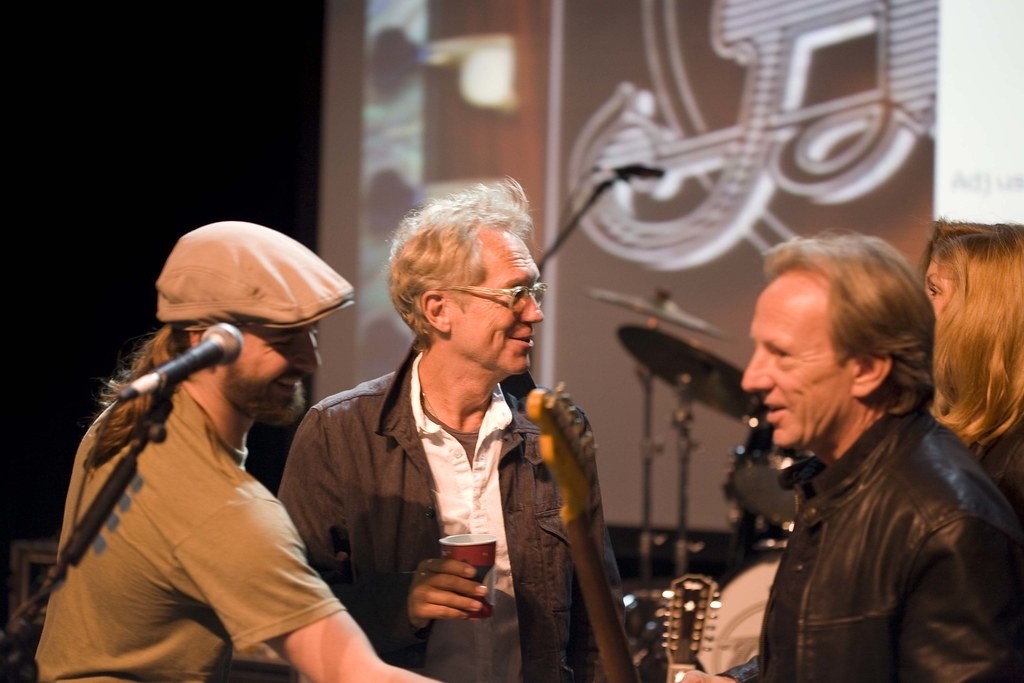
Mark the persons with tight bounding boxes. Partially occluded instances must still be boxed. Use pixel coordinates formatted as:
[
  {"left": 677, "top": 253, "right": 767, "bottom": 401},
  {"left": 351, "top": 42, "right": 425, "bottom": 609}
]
[
  {"left": 33, "top": 220, "right": 439, "bottom": 683},
  {"left": 916, "top": 219, "right": 1024, "bottom": 518},
  {"left": 680, "top": 234, "right": 1024, "bottom": 683},
  {"left": 278, "top": 179, "right": 625, "bottom": 683}
]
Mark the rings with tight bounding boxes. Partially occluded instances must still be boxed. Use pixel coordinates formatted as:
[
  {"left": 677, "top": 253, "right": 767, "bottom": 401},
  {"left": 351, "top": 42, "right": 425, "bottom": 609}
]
[{"left": 421, "top": 559, "right": 433, "bottom": 584}]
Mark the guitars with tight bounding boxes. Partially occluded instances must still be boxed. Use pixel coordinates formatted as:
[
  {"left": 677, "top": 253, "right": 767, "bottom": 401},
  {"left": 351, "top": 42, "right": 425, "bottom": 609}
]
[{"left": 655, "top": 574, "right": 722, "bottom": 683}]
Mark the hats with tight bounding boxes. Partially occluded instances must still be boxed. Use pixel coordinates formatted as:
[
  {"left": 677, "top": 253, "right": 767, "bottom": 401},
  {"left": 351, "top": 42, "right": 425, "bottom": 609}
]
[{"left": 155, "top": 221, "right": 356, "bottom": 331}]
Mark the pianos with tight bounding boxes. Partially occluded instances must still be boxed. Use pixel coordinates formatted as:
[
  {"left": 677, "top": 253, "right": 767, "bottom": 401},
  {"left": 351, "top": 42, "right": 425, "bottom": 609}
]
[{"left": 605, "top": 522, "right": 763, "bottom": 596}]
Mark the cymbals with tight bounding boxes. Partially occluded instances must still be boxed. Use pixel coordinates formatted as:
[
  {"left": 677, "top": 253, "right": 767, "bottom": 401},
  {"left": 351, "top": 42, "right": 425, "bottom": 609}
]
[
  {"left": 615, "top": 320, "right": 768, "bottom": 424},
  {"left": 586, "top": 287, "right": 730, "bottom": 341}
]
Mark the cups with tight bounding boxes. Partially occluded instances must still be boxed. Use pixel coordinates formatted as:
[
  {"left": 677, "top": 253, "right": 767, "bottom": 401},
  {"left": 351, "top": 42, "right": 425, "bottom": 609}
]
[{"left": 437, "top": 533, "right": 499, "bottom": 620}]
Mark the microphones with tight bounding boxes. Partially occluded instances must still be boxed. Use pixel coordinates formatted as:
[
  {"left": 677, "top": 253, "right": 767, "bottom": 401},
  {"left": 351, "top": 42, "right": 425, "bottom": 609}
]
[
  {"left": 597, "top": 162, "right": 664, "bottom": 180},
  {"left": 118, "top": 322, "right": 243, "bottom": 405}
]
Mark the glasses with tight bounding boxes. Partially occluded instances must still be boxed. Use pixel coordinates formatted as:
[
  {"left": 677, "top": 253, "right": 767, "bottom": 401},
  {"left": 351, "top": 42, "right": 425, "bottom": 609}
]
[{"left": 430, "top": 282, "right": 548, "bottom": 312}]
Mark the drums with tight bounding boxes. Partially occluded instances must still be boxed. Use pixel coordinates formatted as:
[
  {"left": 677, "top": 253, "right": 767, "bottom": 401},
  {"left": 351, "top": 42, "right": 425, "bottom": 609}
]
[{"left": 722, "top": 418, "right": 831, "bottom": 543}]
